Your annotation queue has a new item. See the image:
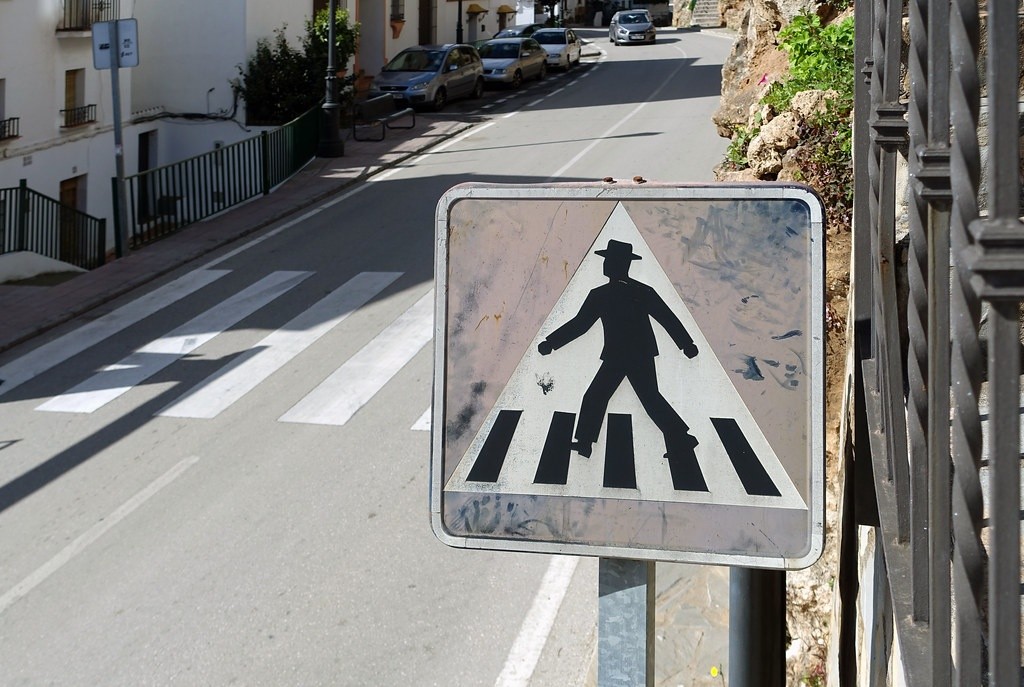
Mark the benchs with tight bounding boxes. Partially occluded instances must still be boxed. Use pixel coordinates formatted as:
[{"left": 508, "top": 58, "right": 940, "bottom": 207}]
[{"left": 351, "top": 93, "right": 416, "bottom": 142}]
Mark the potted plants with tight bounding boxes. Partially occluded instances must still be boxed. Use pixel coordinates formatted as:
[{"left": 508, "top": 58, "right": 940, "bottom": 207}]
[{"left": 303, "top": 2, "right": 361, "bottom": 79}]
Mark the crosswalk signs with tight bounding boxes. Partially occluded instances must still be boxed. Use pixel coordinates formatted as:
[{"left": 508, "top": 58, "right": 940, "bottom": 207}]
[{"left": 431, "top": 180, "right": 827, "bottom": 572}]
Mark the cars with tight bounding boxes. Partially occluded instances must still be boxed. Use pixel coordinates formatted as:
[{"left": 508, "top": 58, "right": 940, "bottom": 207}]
[
  {"left": 609, "top": 10, "right": 658, "bottom": 44},
  {"left": 369, "top": 44, "right": 485, "bottom": 111},
  {"left": 477, "top": 24, "right": 582, "bottom": 87}
]
